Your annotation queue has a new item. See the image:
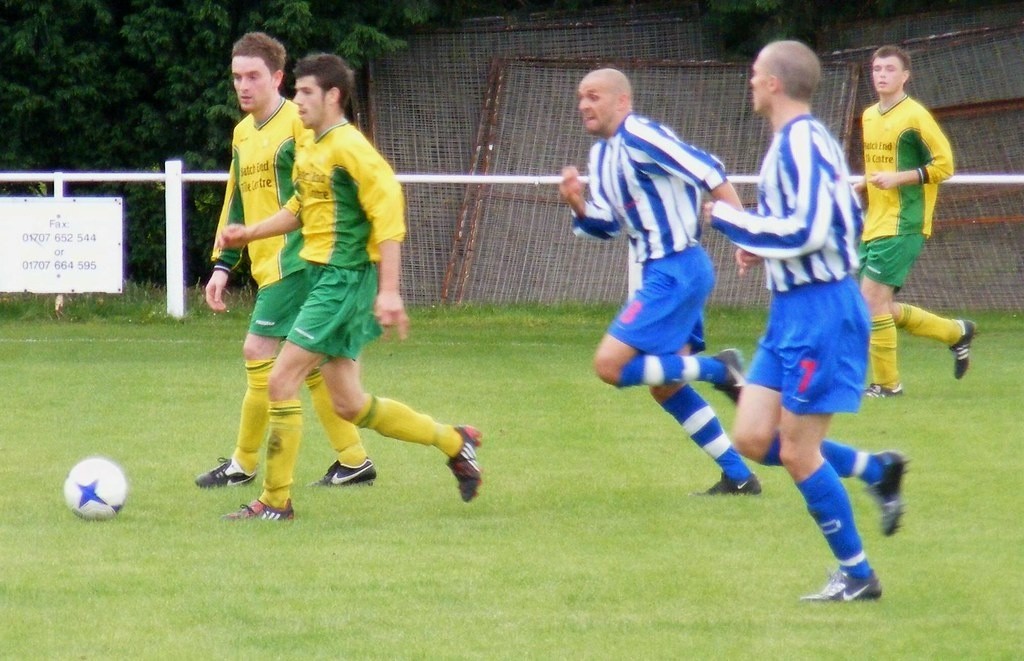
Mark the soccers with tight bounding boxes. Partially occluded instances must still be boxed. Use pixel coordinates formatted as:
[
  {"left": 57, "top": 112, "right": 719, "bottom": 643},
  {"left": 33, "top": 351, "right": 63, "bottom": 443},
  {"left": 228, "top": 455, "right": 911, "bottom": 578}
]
[{"left": 62, "top": 458, "right": 128, "bottom": 522}]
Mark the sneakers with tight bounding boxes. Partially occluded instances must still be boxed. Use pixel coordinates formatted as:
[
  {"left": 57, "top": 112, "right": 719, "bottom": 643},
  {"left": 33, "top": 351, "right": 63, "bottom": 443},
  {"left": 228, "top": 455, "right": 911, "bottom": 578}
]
[
  {"left": 948, "top": 320, "right": 976, "bottom": 379},
  {"left": 306, "top": 457, "right": 376, "bottom": 487},
  {"left": 862, "top": 383, "right": 903, "bottom": 399},
  {"left": 712, "top": 349, "right": 746, "bottom": 404},
  {"left": 864, "top": 450, "right": 909, "bottom": 537},
  {"left": 800, "top": 568, "right": 881, "bottom": 605},
  {"left": 445, "top": 425, "right": 482, "bottom": 502},
  {"left": 194, "top": 457, "right": 257, "bottom": 487},
  {"left": 222, "top": 499, "right": 294, "bottom": 522},
  {"left": 690, "top": 471, "right": 762, "bottom": 497}
]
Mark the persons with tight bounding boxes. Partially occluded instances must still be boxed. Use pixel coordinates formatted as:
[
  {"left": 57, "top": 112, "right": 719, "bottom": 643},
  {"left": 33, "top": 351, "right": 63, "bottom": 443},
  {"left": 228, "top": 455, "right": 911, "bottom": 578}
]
[
  {"left": 847, "top": 44, "right": 977, "bottom": 400},
  {"left": 194, "top": 33, "right": 375, "bottom": 488},
  {"left": 703, "top": 39, "right": 908, "bottom": 604},
  {"left": 561, "top": 67, "right": 761, "bottom": 497},
  {"left": 221, "top": 52, "right": 481, "bottom": 521}
]
